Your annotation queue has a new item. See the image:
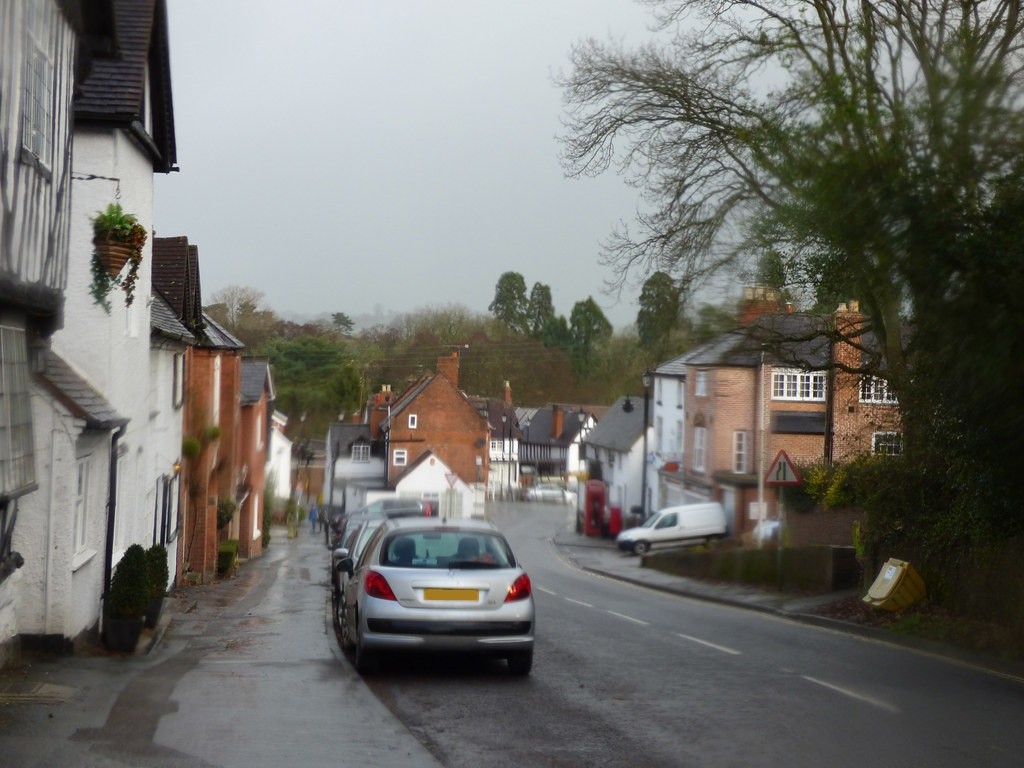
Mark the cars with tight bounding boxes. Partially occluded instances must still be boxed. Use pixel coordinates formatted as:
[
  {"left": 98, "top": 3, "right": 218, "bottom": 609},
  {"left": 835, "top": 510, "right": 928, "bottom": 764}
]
[
  {"left": 335, "top": 516, "right": 535, "bottom": 676},
  {"left": 333, "top": 494, "right": 461, "bottom": 607}
]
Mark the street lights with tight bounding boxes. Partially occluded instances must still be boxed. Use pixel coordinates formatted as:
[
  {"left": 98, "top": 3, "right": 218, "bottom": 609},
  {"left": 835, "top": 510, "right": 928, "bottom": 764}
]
[
  {"left": 640, "top": 369, "right": 653, "bottom": 524},
  {"left": 500, "top": 411, "right": 509, "bottom": 461},
  {"left": 578, "top": 406, "right": 586, "bottom": 442}
]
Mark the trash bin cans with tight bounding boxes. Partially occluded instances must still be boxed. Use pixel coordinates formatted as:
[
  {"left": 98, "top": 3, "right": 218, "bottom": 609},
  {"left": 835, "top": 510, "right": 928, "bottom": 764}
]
[{"left": 861, "top": 557, "right": 926, "bottom": 613}]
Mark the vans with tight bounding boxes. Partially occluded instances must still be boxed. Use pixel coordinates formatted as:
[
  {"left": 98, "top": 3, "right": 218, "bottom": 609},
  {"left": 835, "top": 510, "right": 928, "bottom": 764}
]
[{"left": 616, "top": 502, "right": 729, "bottom": 555}]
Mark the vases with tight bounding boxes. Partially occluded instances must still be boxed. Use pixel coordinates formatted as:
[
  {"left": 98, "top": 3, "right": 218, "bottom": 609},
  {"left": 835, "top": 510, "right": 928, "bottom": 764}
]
[{"left": 95, "top": 229, "right": 131, "bottom": 281}]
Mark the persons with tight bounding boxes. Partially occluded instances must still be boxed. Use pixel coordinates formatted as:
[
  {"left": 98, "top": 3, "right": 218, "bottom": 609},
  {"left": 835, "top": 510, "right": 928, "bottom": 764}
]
[{"left": 309, "top": 504, "right": 319, "bottom": 532}]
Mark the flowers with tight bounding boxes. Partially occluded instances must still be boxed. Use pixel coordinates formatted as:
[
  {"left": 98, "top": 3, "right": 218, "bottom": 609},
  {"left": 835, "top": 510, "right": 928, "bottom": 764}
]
[{"left": 87, "top": 202, "right": 148, "bottom": 317}]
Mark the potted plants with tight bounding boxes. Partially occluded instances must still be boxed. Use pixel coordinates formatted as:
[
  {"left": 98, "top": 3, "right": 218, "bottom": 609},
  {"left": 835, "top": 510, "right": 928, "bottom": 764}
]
[
  {"left": 100, "top": 542, "right": 153, "bottom": 654},
  {"left": 144, "top": 544, "right": 169, "bottom": 632}
]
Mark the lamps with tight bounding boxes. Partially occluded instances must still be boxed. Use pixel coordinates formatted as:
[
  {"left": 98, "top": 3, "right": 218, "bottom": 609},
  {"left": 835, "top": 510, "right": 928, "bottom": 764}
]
[{"left": 166, "top": 455, "right": 181, "bottom": 486}]
